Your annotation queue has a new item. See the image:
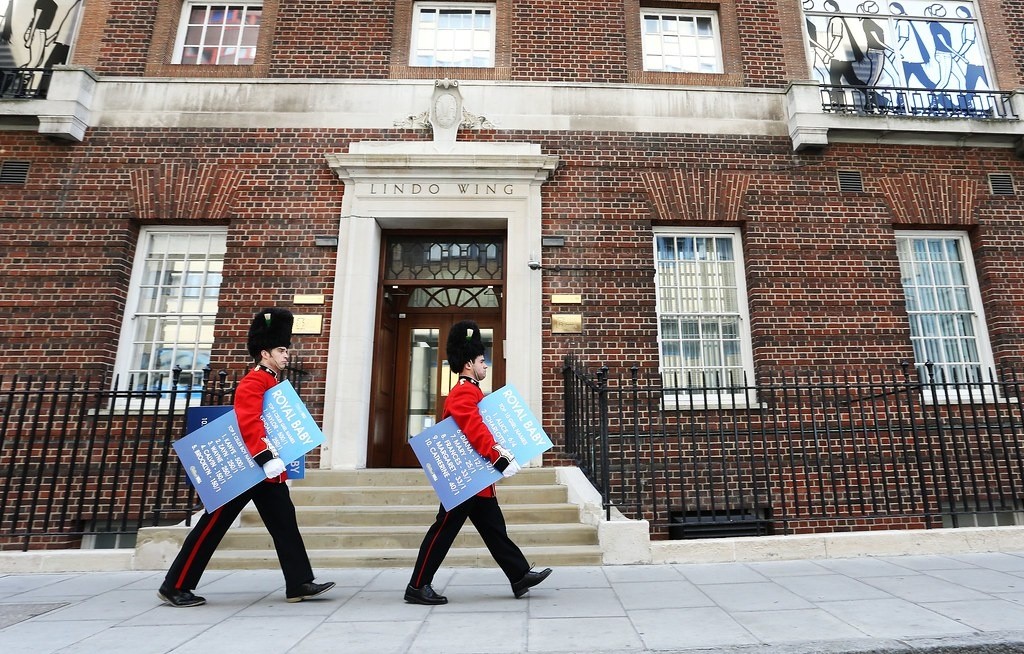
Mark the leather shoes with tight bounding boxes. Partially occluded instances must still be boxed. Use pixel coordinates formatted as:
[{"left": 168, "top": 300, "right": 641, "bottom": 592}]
[
  {"left": 403, "top": 584, "right": 448, "bottom": 605},
  {"left": 156, "top": 580, "right": 206, "bottom": 608},
  {"left": 285, "top": 581, "right": 336, "bottom": 603},
  {"left": 510, "top": 561, "right": 553, "bottom": 599}
]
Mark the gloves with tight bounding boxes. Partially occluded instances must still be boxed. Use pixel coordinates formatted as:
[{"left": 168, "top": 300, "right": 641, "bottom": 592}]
[
  {"left": 263, "top": 458, "right": 287, "bottom": 479},
  {"left": 502, "top": 459, "right": 521, "bottom": 479}
]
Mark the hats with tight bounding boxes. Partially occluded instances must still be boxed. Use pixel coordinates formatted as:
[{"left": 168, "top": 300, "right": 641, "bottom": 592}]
[
  {"left": 446, "top": 320, "right": 484, "bottom": 374},
  {"left": 247, "top": 308, "right": 293, "bottom": 362}
]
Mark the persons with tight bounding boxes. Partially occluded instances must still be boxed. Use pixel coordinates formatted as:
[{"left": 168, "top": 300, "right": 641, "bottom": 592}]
[
  {"left": 404, "top": 321, "right": 552, "bottom": 605},
  {"left": 158, "top": 308, "right": 335, "bottom": 608}
]
